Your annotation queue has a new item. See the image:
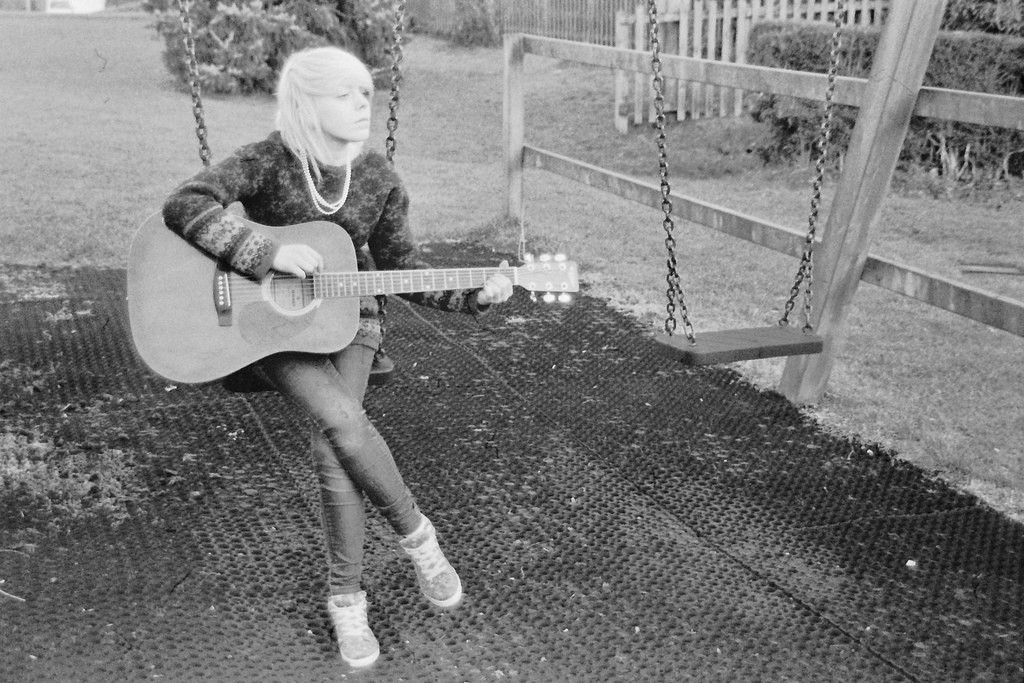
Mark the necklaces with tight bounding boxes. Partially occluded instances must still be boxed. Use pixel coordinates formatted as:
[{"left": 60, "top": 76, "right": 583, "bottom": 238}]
[{"left": 296, "top": 145, "right": 353, "bottom": 215}]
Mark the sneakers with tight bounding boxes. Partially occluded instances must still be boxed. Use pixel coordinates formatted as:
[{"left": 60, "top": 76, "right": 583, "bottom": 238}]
[
  {"left": 326, "top": 589, "right": 380, "bottom": 667},
  {"left": 398, "top": 512, "right": 462, "bottom": 608}
]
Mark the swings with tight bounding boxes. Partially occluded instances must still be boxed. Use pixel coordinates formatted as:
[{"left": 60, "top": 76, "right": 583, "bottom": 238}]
[
  {"left": 647, "top": 1, "right": 848, "bottom": 365},
  {"left": 177, "top": 0, "right": 405, "bottom": 392}
]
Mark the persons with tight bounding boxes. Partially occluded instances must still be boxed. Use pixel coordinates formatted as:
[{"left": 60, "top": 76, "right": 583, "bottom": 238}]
[{"left": 158, "top": 48, "right": 463, "bottom": 668}]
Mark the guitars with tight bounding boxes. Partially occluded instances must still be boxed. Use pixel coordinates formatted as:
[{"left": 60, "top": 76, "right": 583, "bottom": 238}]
[{"left": 126, "top": 208, "right": 580, "bottom": 384}]
